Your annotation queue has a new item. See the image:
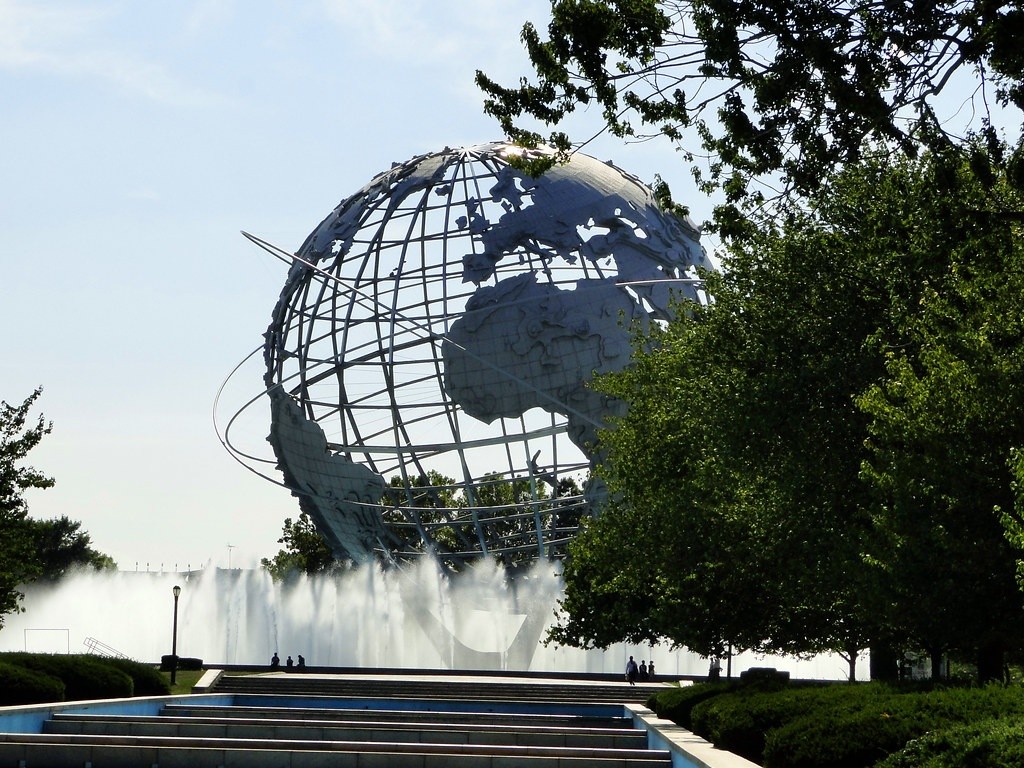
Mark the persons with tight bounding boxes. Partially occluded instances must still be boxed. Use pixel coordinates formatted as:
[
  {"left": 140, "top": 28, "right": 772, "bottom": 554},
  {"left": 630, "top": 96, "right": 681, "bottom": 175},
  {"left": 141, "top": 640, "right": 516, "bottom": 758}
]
[
  {"left": 626, "top": 656, "right": 638, "bottom": 685},
  {"left": 639, "top": 660, "right": 646, "bottom": 673},
  {"left": 286, "top": 656, "right": 293, "bottom": 667},
  {"left": 649, "top": 661, "right": 654, "bottom": 675},
  {"left": 296, "top": 655, "right": 305, "bottom": 667},
  {"left": 271, "top": 653, "right": 280, "bottom": 666},
  {"left": 708, "top": 655, "right": 721, "bottom": 679}
]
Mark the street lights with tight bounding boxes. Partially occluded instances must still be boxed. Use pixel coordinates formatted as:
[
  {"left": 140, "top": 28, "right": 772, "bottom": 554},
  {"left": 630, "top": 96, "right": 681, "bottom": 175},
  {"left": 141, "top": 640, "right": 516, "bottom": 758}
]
[
  {"left": 170, "top": 585, "right": 181, "bottom": 685},
  {"left": 228, "top": 545, "right": 232, "bottom": 569}
]
